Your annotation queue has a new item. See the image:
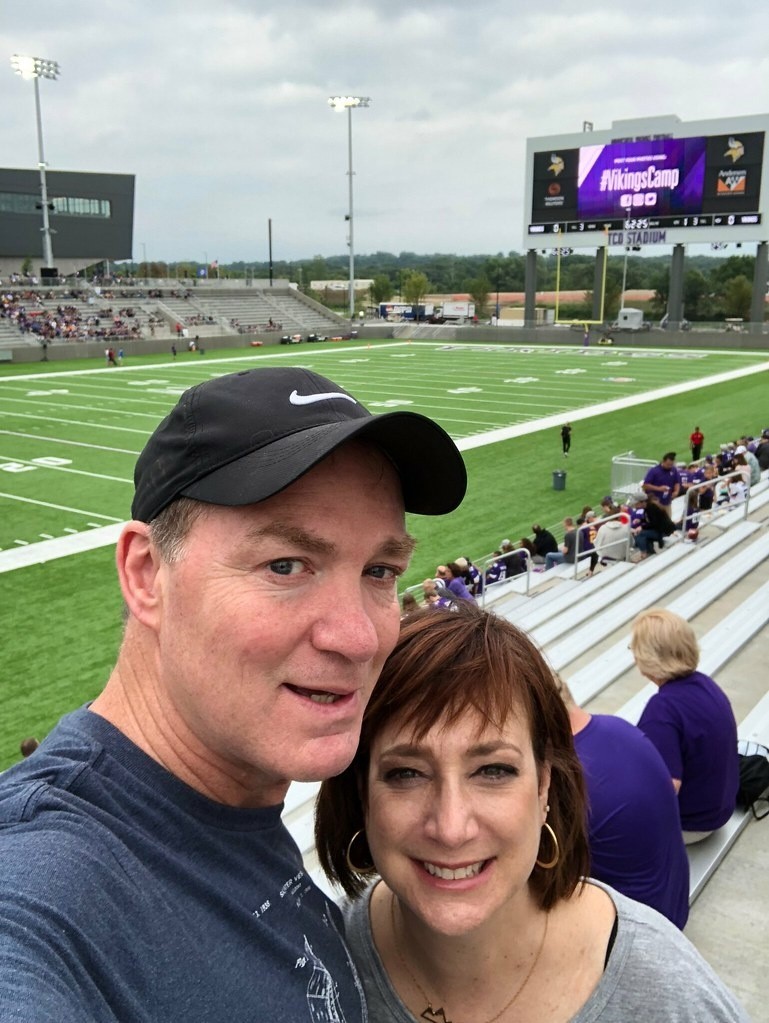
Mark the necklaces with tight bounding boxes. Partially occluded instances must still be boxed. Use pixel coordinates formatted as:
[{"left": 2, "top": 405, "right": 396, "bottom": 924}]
[{"left": 390, "top": 893, "right": 548, "bottom": 1023}]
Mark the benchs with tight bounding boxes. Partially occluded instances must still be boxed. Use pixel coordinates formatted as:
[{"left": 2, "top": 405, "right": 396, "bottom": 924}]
[{"left": 282, "top": 468, "right": 769, "bottom": 907}]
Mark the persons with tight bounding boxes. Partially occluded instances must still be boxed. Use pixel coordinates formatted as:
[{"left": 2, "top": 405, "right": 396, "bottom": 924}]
[
  {"left": 171, "top": 343, "right": 176, "bottom": 359},
  {"left": 314, "top": 603, "right": 753, "bottom": 1022},
  {"left": 546, "top": 671, "right": 689, "bottom": 936},
  {"left": 626, "top": 609, "right": 737, "bottom": 848},
  {"left": 688, "top": 426, "right": 705, "bottom": 461},
  {"left": 0, "top": 367, "right": 465, "bottom": 1023},
  {"left": 560, "top": 423, "right": 572, "bottom": 457},
  {"left": 0, "top": 270, "right": 282, "bottom": 366},
  {"left": 401, "top": 428, "right": 769, "bottom": 613}
]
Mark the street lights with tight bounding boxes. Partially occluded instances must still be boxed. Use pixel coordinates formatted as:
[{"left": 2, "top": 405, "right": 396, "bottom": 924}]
[
  {"left": 327, "top": 94, "right": 372, "bottom": 321},
  {"left": 11, "top": 52, "right": 64, "bottom": 286}
]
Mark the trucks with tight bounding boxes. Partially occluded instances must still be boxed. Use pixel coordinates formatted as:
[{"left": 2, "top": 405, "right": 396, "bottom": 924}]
[
  {"left": 434, "top": 300, "right": 476, "bottom": 322},
  {"left": 377, "top": 300, "right": 434, "bottom": 322}
]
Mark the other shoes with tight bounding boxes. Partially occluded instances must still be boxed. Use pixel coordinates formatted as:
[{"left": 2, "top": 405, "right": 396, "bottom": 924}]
[{"left": 564, "top": 452, "right": 568, "bottom": 458}]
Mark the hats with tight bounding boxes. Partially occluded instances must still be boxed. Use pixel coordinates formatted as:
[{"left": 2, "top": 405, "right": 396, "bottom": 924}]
[
  {"left": 631, "top": 492, "right": 647, "bottom": 504},
  {"left": 131, "top": 367, "right": 468, "bottom": 522},
  {"left": 435, "top": 565, "right": 452, "bottom": 578},
  {"left": 735, "top": 445, "right": 746, "bottom": 455},
  {"left": 499, "top": 540, "right": 510, "bottom": 549},
  {"left": 585, "top": 511, "right": 596, "bottom": 520}
]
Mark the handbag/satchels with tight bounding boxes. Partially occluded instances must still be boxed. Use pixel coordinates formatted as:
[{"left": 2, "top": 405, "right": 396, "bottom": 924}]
[{"left": 735, "top": 740, "right": 769, "bottom": 820}]
